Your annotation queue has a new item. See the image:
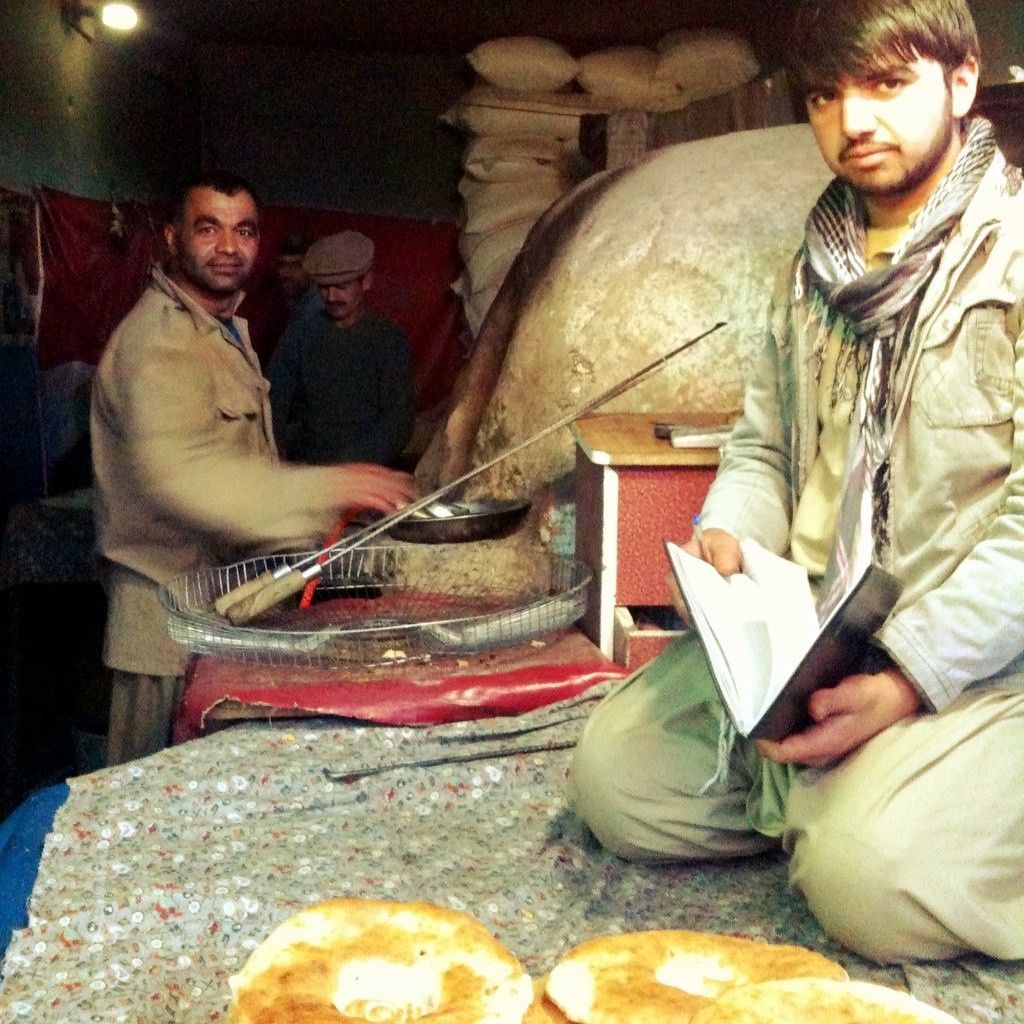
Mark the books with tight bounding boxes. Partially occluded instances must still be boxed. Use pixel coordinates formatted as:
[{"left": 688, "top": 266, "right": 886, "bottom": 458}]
[{"left": 662, "top": 539, "right": 905, "bottom": 738}]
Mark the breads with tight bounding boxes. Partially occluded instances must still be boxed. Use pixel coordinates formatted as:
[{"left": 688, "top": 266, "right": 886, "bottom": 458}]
[{"left": 217, "top": 899, "right": 960, "bottom": 1024}]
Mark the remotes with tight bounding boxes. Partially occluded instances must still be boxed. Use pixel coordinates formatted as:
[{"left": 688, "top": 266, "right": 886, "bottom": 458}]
[{"left": 655, "top": 422, "right": 738, "bottom": 448}]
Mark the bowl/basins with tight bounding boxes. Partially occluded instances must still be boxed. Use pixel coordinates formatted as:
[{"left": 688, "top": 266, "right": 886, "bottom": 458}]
[{"left": 383, "top": 495, "right": 534, "bottom": 545}]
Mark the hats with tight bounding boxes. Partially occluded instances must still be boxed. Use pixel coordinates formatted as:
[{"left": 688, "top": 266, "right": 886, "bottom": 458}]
[{"left": 302, "top": 231, "right": 374, "bottom": 285}]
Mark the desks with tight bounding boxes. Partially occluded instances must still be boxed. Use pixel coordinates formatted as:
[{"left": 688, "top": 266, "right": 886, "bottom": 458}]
[{"left": 175, "top": 590, "right": 618, "bottom": 745}]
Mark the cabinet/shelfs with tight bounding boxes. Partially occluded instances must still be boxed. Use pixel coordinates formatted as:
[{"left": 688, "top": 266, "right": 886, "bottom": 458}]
[{"left": 565, "top": 406, "right": 740, "bottom": 668}]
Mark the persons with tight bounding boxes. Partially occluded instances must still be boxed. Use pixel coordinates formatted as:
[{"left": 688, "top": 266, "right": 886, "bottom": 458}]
[
  {"left": 89, "top": 171, "right": 420, "bottom": 771},
  {"left": 268, "top": 230, "right": 416, "bottom": 466},
  {"left": 570, "top": 0, "right": 1024, "bottom": 970}
]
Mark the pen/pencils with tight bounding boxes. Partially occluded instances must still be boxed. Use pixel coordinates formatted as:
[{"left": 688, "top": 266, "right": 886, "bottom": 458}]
[{"left": 693, "top": 515, "right": 712, "bottom": 564}]
